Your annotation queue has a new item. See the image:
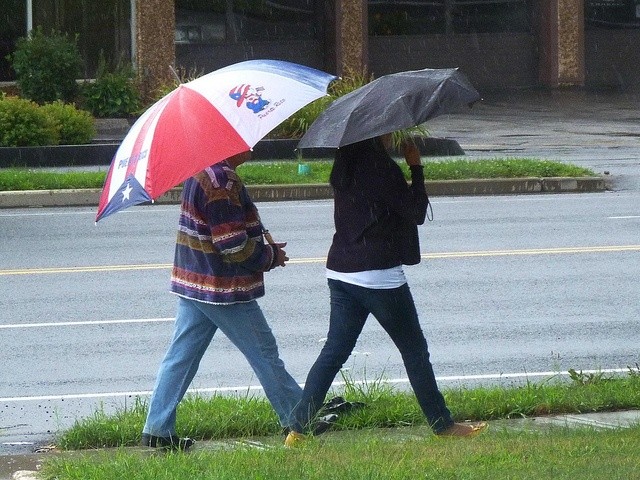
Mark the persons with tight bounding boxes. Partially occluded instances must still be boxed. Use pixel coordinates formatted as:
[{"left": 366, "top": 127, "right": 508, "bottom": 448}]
[
  {"left": 284, "top": 132, "right": 489, "bottom": 449},
  {"left": 141, "top": 148, "right": 339, "bottom": 451}
]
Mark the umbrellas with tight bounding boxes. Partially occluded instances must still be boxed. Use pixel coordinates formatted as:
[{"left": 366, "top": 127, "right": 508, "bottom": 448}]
[
  {"left": 295, "top": 66, "right": 486, "bottom": 147},
  {"left": 94, "top": 58, "right": 343, "bottom": 243}
]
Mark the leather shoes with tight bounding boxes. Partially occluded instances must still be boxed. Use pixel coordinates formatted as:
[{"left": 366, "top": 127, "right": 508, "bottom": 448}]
[
  {"left": 436, "top": 422, "right": 491, "bottom": 441},
  {"left": 283, "top": 414, "right": 339, "bottom": 435},
  {"left": 284, "top": 431, "right": 308, "bottom": 448},
  {"left": 141, "top": 432, "right": 193, "bottom": 450}
]
